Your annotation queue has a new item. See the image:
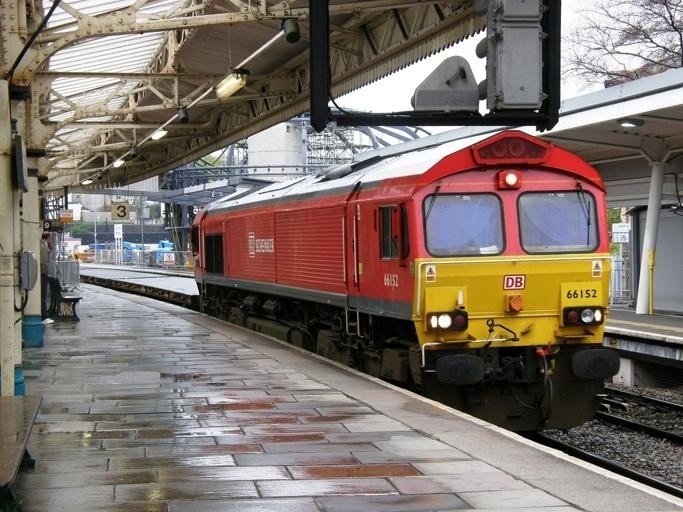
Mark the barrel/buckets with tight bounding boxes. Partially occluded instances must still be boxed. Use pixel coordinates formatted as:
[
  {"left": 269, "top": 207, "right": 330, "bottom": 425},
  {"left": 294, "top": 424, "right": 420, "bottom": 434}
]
[
  {"left": 21, "top": 315, "right": 45, "bottom": 348},
  {"left": 14, "top": 363, "right": 25, "bottom": 395}
]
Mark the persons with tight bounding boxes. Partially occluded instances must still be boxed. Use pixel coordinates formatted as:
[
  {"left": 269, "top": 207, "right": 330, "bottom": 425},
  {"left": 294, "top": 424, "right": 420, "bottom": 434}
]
[{"left": 41, "top": 231, "right": 54, "bottom": 324}]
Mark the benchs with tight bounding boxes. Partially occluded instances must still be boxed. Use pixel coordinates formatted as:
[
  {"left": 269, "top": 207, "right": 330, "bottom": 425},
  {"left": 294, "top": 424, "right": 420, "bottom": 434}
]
[{"left": 48, "top": 277, "right": 83, "bottom": 322}]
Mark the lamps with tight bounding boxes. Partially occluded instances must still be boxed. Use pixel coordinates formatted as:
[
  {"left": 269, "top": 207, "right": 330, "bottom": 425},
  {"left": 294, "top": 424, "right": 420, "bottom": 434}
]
[
  {"left": 214, "top": 69, "right": 250, "bottom": 102},
  {"left": 130, "top": 147, "right": 137, "bottom": 158},
  {"left": 178, "top": 109, "right": 190, "bottom": 123},
  {"left": 97, "top": 171, "right": 103, "bottom": 180},
  {"left": 282, "top": 17, "right": 300, "bottom": 42}
]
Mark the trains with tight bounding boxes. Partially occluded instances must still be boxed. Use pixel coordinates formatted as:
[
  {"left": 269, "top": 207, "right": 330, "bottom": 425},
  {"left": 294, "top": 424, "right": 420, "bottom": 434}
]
[{"left": 188, "top": 130, "right": 620, "bottom": 433}]
